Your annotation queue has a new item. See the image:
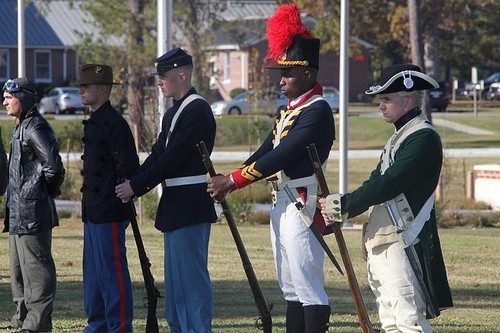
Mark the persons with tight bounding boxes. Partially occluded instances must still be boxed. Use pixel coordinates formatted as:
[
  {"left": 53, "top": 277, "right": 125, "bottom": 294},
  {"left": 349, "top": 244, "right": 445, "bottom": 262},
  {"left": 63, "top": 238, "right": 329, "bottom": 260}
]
[
  {"left": 115, "top": 47, "right": 217, "bottom": 333},
  {"left": 206, "top": 4, "right": 336, "bottom": 333},
  {"left": 317, "top": 64, "right": 455, "bottom": 333},
  {"left": 70, "top": 63, "right": 141, "bottom": 333},
  {"left": 0, "top": 77, "right": 65, "bottom": 333}
]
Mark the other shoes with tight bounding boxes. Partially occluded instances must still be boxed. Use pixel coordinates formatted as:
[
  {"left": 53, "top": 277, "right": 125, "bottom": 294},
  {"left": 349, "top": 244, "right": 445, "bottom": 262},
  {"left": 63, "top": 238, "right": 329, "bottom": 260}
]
[
  {"left": 10, "top": 329, "right": 39, "bottom": 333},
  {"left": 0, "top": 325, "right": 13, "bottom": 330}
]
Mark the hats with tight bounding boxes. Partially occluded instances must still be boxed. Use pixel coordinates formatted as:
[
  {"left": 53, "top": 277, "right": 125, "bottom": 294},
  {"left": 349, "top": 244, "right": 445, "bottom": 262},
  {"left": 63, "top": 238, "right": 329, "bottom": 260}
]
[
  {"left": 365, "top": 63, "right": 440, "bottom": 95},
  {"left": 2, "top": 76, "right": 35, "bottom": 121},
  {"left": 148, "top": 47, "right": 193, "bottom": 76},
  {"left": 72, "top": 64, "right": 124, "bottom": 87},
  {"left": 261, "top": 4, "right": 320, "bottom": 70}
]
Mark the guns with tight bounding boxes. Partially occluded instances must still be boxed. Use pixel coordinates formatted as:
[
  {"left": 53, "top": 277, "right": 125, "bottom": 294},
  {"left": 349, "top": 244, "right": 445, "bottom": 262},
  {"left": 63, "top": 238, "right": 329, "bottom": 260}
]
[
  {"left": 112, "top": 151, "right": 160, "bottom": 333},
  {"left": 306, "top": 142, "right": 375, "bottom": 333},
  {"left": 194, "top": 140, "right": 274, "bottom": 333}
]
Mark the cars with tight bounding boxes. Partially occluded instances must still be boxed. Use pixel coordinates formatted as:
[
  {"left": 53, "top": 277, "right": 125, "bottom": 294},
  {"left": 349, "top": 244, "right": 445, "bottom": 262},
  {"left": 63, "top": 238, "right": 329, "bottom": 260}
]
[
  {"left": 489, "top": 82, "right": 500, "bottom": 100},
  {"left": 210, "top": 89, "right": 288, "bottom": 116},
  {"left": 428, "top": 83, "right": 450, "bottom": 111},
  {"left": 38, "top": 87, "right": 89, "bottom": 114},
  {"left": 455, "top": 72, "right": 500, "bottom": 96},
  {"left": 322, "top": 86, "right": 339, "bottom": 112}
]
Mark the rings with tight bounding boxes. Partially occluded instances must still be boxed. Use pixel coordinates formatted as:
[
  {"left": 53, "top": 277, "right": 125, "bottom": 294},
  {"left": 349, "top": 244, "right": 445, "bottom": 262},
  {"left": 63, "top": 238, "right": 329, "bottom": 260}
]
[{"left": 115, "top": 190, "right": 119, "bottom": 194}]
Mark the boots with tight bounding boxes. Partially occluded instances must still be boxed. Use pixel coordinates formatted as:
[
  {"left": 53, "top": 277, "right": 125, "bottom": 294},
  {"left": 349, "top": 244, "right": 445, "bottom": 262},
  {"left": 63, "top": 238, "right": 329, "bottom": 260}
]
[
  {"left": 286, "top": 300, "right": 304, "bottom": 333},
  {"left": 303, "top": 305, "right": 331, "bottom": 333}
]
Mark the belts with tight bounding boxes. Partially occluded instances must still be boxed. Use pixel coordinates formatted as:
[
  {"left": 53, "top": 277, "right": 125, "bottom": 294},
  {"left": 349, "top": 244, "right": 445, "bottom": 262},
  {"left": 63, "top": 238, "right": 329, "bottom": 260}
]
[
  {"left": 162, "top": 174, "right": 208, "bottom": 188},
  {"left": 269, "top": 174, "right": 320, "bottom": 192}
]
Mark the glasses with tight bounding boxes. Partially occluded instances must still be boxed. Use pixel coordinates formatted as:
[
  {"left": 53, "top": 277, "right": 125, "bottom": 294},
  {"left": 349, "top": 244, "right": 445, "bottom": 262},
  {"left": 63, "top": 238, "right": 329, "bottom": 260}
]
[{"left": 2, "top": 80, "right": 37, "bottom": 95}]
